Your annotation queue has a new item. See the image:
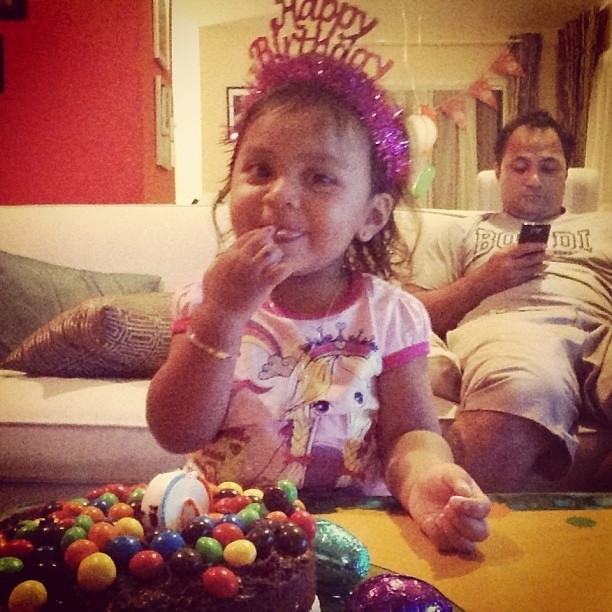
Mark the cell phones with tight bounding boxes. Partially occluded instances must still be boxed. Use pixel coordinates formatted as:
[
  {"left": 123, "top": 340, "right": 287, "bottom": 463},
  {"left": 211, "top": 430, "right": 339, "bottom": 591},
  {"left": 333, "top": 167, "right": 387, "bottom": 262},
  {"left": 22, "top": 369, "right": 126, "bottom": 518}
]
[{"left": 518, "top": 222, "right": 551, "bottom": 243}]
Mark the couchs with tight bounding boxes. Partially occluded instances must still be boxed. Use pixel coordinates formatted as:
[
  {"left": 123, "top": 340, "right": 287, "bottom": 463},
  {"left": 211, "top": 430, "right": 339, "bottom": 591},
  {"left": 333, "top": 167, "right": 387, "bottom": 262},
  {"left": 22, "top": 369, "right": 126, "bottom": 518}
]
[{"left": 1, "top": 198, "right": 611, "bottom": 480}]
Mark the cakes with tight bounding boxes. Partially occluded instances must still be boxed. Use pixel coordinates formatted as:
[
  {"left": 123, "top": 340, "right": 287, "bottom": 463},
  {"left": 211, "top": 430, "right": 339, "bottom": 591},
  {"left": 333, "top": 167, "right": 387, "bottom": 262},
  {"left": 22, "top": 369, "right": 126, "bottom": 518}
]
[{"left": 0, "top": 472, "right": 318, "bottom": 612}]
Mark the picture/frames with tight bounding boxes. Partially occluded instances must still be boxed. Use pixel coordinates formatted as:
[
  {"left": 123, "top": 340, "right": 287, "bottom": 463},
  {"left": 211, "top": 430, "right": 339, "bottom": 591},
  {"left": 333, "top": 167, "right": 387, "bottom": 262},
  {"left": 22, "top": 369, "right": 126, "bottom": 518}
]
[
  {"left": 152, "top": 0, "right": 171, "bottom": 70},
  {"left": 154, "top": 74, "right": 172, "bottom": 172},
  {"left": 226, "top": 86, "right": 258, "bottom": 142}
]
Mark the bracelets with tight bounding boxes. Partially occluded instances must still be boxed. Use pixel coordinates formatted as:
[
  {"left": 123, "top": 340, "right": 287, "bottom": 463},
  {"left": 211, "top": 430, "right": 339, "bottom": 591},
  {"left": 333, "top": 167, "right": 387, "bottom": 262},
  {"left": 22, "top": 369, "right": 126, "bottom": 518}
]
[{"left": 184, "top": 325, "right": 244, "bottom": 362}]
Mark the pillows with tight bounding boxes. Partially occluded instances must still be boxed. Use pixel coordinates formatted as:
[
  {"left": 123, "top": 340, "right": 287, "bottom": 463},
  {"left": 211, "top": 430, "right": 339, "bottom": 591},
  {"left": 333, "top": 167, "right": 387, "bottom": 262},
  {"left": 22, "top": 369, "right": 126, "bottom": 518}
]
[
  {"left": 3, "top": 295, "right": 174, "bottom": 375},
  {"left": 1, "top": 249, "right": 160, "bottom": 355}
]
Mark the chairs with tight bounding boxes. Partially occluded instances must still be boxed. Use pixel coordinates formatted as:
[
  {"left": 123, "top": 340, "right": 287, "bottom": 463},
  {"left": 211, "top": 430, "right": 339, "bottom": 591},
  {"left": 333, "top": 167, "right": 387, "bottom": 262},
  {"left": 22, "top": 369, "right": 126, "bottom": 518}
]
[{"left": 476, "top": 165, "right": 600, "bottom": 212}]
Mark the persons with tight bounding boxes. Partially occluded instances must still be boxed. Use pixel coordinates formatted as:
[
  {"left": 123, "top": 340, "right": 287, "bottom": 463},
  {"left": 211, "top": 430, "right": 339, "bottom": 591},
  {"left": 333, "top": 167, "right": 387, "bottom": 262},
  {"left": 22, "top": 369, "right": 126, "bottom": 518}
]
[
  {"left": 402, "top": 110, "right": 610, "bottom": 492},
  {"left": 147, "top": 77, "right": 490, "bottom": 553}
]
